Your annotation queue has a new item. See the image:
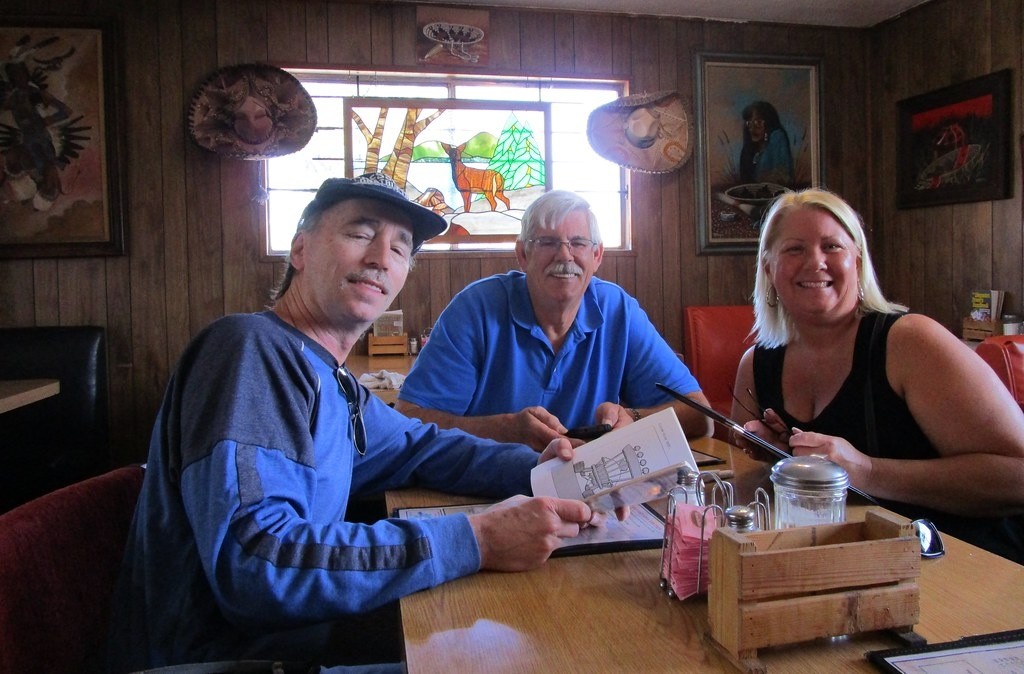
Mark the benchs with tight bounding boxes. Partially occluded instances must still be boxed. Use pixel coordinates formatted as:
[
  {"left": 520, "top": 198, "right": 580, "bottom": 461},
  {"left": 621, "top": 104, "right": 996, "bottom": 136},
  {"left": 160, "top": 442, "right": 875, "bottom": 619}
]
[{"left": 0, "top": 325, "right": 114, "bottom": 502}]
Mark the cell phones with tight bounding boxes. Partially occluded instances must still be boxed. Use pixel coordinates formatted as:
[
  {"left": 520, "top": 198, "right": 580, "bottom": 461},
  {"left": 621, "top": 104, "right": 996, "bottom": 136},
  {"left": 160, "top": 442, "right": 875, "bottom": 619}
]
[{"left": 563, "top": 423, "right": 612, "bottom": 439}]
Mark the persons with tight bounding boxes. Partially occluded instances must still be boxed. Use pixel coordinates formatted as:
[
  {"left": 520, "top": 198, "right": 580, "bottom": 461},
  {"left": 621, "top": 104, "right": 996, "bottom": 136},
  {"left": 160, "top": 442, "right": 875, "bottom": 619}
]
[
  {"left": 103, "top": 171, "right": 630, "bottom": 674},
  {"left": 728, "top": 180, "right": 1024, "bottom": 565},
  {"left": 393, "top": 187, "right": 714, "bottom": 449}
]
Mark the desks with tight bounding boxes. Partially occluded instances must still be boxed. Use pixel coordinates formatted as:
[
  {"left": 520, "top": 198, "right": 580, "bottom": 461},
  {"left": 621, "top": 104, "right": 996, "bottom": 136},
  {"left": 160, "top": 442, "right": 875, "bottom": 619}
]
[
  {"left": 384, "top": 439, "right": 1024, "bottom": 674},
  {"left": 344, "top": 355, "right": 419, "bottom": 408}
]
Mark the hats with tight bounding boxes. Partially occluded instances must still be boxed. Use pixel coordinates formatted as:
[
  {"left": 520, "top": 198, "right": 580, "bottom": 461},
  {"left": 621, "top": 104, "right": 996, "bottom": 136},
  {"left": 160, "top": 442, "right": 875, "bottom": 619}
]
[
  {"left": 587, "top": 91, "right": 694, "bottom": 173},
  {"left": 316, "top": 172, "right": 447, "bottom": 241},
  {"left": 188, "top": 62, "right": 316, "bottom": 160}
]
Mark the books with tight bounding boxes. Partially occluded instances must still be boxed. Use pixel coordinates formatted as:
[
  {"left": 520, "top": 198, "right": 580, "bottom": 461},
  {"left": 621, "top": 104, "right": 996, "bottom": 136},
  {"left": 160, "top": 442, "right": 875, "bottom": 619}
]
[{"left": 530, "top": 406, "right": 704, "bottom": 514}]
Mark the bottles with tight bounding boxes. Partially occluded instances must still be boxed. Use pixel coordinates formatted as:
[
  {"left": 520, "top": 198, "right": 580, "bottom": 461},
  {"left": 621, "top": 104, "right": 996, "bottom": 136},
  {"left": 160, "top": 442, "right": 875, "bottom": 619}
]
[
  {"left": 411, "top": 338, "right": 417, "bottom": 353},
  {"left": 673, "top": 471, "right": 705, "bottom": 506},
  {"left": 770, "top": 455, "right": 850, "bottom": 529},
  {"left": 1002, "top": 315, "right": 1018, "bottom": 335},
  {"left": 726, "top": 505, "right": 761, "bottom": 533}
]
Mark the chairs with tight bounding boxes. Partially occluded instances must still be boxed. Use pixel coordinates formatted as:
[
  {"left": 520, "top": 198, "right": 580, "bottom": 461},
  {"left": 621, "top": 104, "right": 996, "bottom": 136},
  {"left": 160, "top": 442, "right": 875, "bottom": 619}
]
[
  {"left": 0, "top": 468, "right": 139, "bottom": 674},
  {"left": 685, "top": 305, "right": 758, "bottom": 436}
]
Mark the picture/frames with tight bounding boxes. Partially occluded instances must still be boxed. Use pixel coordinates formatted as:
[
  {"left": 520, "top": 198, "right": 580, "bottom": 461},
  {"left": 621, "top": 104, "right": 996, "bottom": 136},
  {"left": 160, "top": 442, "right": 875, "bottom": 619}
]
[
  {"left": 892, "top": 66, "right": 1014, "bottom": 213},
  {"left": 0, "top": 9, "right": 127, "bottom": 259},
  {"left": 693, "top": 52, "right": 830, "bottom": 254}
]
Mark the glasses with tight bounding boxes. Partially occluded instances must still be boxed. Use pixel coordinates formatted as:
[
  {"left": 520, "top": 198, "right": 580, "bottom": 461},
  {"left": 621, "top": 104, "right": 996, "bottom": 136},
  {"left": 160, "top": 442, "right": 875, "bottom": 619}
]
[
  {"left": 525, "top": 238, "right": 595, "bottom": 255},
  {"left": 336, "top": 367, "right": 367, "bottom": 456}
]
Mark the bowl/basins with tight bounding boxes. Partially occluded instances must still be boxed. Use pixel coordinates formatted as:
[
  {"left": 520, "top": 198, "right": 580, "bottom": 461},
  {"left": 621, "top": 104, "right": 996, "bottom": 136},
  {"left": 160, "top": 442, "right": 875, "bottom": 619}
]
[
  {"left": 423, "top": 22, "right": 484, "bottom": 44},
  {"left": 725, "top": 183, "right": 791, "bottom": 205}
]
